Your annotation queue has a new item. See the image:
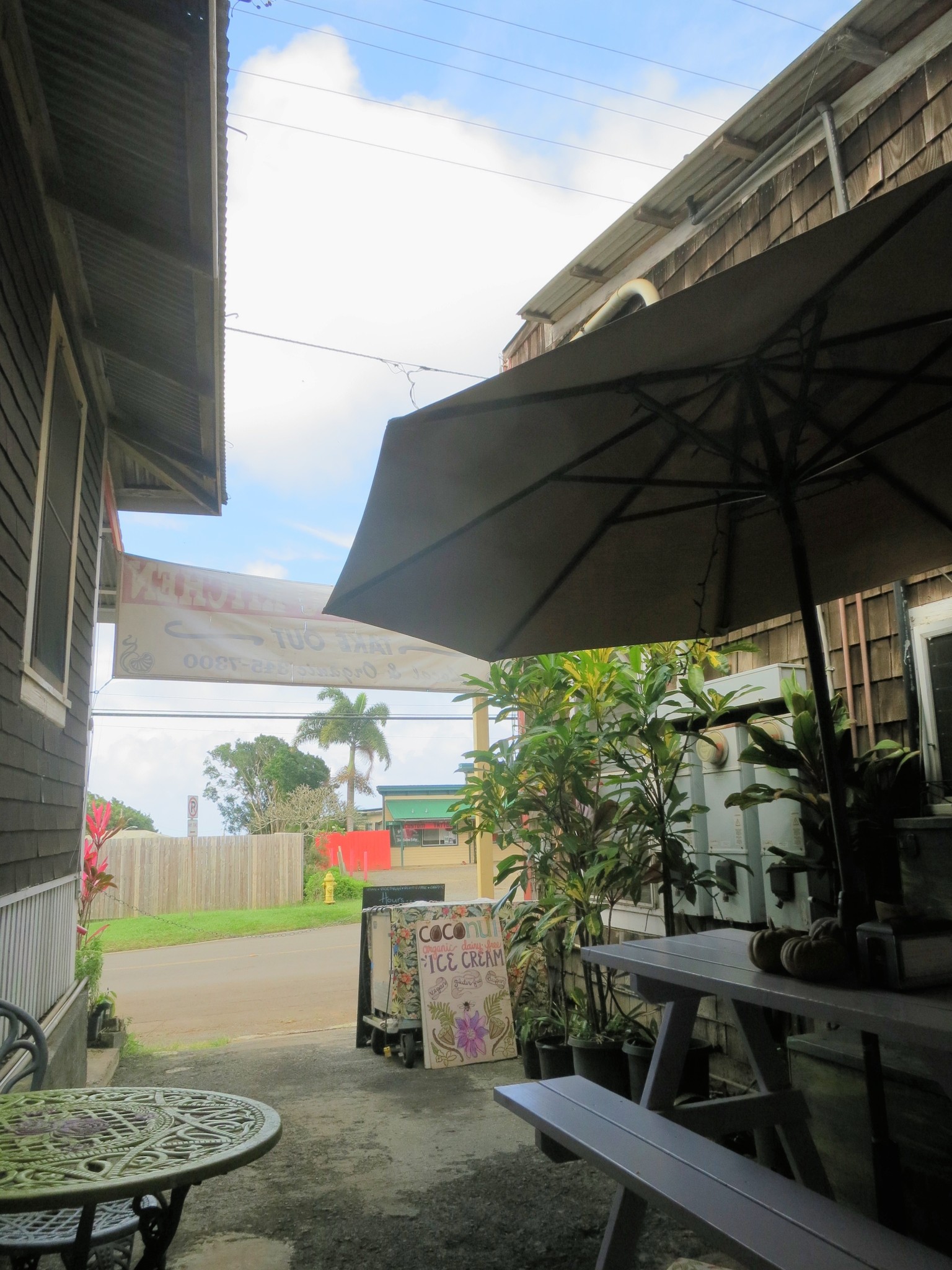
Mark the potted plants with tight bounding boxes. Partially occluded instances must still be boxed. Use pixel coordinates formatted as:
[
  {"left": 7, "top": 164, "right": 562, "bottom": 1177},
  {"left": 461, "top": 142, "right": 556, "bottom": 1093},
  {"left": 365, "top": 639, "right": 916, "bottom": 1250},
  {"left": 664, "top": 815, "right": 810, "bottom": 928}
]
[{"left": 433, "top": 627, "right": 755, "bottom": 1110}]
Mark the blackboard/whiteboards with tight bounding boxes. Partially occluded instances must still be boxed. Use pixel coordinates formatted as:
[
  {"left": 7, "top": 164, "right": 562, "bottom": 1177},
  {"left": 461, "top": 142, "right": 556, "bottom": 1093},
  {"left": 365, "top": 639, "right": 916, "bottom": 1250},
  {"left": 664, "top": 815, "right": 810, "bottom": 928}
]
[
  {"left": 415, "top": 917, "right": 520, "bottom": 1068},
  {"left": 357, "top": 883, "right": 448, "bottom": 1046}
]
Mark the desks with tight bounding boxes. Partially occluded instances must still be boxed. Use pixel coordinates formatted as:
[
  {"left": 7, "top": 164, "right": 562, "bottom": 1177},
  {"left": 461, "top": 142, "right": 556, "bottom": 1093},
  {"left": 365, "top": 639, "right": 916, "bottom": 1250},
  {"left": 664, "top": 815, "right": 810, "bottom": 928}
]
[
  {"left": 581, "top": 929, "right": 952, "bottom": 1270},
  {"left": 0, "top": 1087, "right": 282, "bottom": 1270}
]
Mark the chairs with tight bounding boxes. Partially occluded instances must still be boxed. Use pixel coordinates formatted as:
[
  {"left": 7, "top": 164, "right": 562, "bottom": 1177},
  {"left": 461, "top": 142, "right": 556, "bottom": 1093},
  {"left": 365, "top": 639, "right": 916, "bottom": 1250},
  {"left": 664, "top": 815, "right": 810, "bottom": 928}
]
[{"left": 0, "top": 998, "right": 160, "bottom": 1270}]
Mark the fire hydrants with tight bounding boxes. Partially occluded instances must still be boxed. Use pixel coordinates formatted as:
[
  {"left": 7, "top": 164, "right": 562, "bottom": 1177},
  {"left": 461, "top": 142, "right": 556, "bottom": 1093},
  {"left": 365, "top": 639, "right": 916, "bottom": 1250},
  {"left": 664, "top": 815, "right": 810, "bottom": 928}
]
[{"left": 321, "top": 870, "right": 338, "bottom": 905}]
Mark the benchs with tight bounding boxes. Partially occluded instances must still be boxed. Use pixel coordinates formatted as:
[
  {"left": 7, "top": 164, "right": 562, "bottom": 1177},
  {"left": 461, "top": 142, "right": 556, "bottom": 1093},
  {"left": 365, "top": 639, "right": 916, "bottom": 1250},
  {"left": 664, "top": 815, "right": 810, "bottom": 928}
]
[
  {"left": 492, "top": 1074, "right": 952, "bottom": 1270},
  {"left": 786, "top": 1030, "right": 952, "bottom": 1092}
]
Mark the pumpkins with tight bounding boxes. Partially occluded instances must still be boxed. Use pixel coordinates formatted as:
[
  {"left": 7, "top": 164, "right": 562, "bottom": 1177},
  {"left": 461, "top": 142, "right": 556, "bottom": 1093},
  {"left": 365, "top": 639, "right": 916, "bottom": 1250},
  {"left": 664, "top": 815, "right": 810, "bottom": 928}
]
[{"left": 747, "top": 915, "right": 853, "bottom": 979}]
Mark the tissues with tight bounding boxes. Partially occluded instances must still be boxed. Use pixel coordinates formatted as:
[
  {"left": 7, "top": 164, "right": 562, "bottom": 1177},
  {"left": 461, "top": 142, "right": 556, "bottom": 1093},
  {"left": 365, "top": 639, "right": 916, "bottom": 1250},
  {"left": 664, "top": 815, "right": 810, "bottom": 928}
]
[{"left": 856, "top": 898, "right": 952, "bottom": 992}]
